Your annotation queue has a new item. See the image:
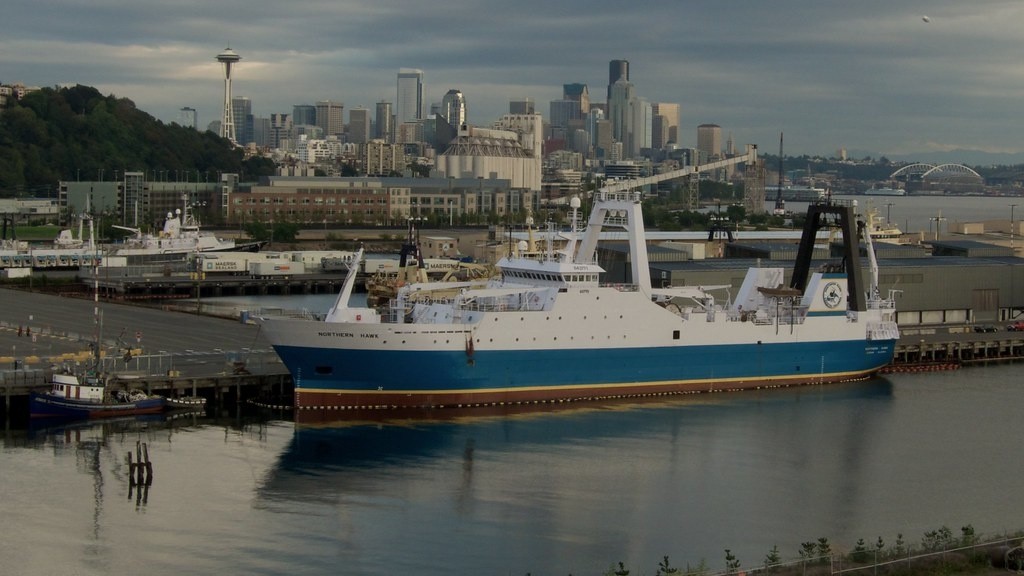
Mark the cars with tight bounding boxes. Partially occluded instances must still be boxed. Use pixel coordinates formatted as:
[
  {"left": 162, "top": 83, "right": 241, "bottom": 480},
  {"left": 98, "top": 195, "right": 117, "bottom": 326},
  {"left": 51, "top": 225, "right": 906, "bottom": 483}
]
[
  {"left": 1006, "top": 319, "right": 1024, "bottom": 331},
  {"left": 974, "top": 323, "right": 998, "bottom": 333}
]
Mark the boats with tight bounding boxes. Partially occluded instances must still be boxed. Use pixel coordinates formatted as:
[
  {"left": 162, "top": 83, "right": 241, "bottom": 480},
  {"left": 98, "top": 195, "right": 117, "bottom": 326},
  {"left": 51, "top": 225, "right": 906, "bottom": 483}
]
[
  {"left": 28, "top": 372, "right": 168, "bottom": 417},
  {"left": 276, "top": 372, "right": 895, "bottom": 478},
  {"left": 248, "top": 191, "right": 905, "bottom": 411},
  {"left": 829, "top": 200, "right": 903, "bottom": 243},
  {"left": 0, "top": 189, "right": 581, "bottom": 324},
  {"left": 27, "top": 405, "right": 177, "bottom": 435},
  {"left": 166, "top": 396, "right": 207, "bottom": 408},
  {"left": 164, "top": 408, "right": 207, "bottom": 423}
]
